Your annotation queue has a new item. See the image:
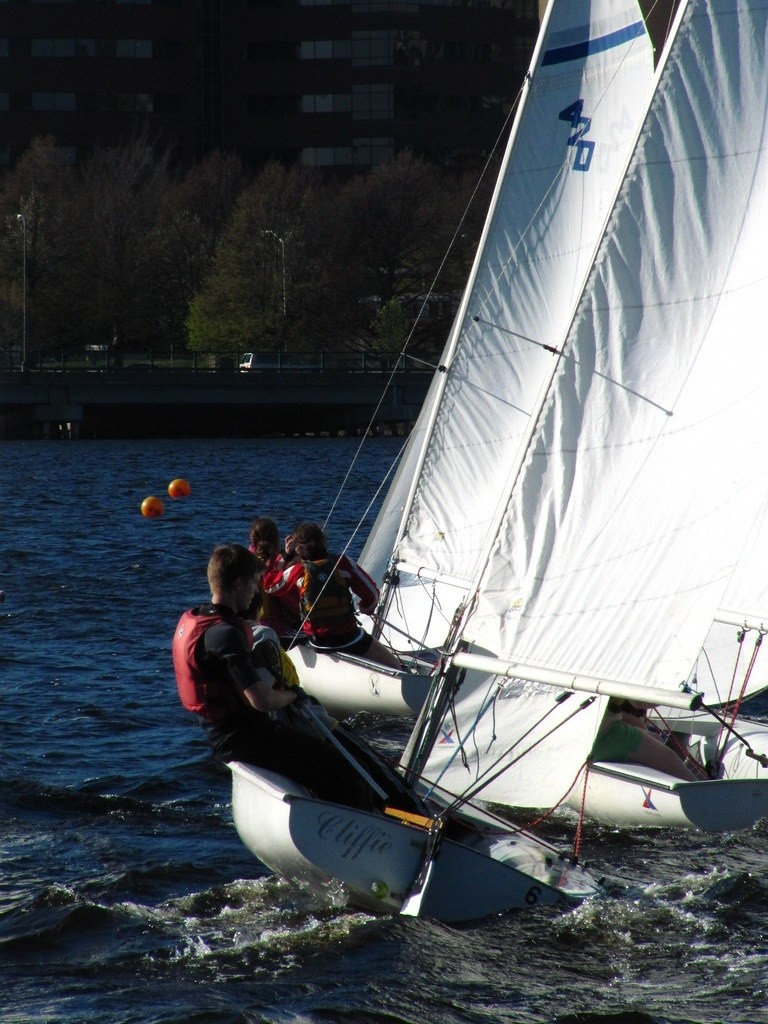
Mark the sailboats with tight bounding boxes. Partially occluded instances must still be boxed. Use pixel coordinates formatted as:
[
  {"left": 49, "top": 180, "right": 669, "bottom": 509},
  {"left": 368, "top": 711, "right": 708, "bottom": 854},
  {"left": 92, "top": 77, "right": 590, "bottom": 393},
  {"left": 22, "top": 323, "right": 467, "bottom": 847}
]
[{"left": 222, "top": 0, "right": 768, "bottom": 925}]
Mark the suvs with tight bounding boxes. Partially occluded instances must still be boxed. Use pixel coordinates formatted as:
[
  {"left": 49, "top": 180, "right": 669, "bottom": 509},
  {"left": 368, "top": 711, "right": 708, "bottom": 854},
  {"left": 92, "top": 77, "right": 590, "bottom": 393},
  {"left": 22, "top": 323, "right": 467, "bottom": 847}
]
[{"left": 238, "top": 349, "right": 321, "bottom": 374}]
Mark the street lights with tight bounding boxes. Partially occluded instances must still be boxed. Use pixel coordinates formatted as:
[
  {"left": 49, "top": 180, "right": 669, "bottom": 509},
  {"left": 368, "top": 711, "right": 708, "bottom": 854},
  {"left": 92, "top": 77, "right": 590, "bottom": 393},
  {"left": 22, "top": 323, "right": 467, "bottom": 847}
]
[
  {"left": 258, "top": 227, "right": 289, "bottom": 353},
  {"left": 16, "top": 212, "right": 29, "bottom": 372}
]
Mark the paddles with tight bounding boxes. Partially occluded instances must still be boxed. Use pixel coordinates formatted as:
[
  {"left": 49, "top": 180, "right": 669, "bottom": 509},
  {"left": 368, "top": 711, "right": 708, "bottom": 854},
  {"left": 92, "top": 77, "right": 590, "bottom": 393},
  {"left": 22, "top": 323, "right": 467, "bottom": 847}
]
[{"left": 268, "top": 664, "right": 446, "bottom": 829}]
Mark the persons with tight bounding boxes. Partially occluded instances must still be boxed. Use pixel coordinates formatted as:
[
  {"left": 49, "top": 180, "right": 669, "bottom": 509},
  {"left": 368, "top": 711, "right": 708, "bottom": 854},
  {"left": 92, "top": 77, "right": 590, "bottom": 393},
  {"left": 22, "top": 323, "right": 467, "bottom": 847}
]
[
  {"left": 172, "top": 544, "right": 430, "bottom": 831},
  {"left": 247, "top": 517, "right": 403, "bottom": 671},
  {"left": 589, "top": 696, "right": 700, "bottom": 782}
]
